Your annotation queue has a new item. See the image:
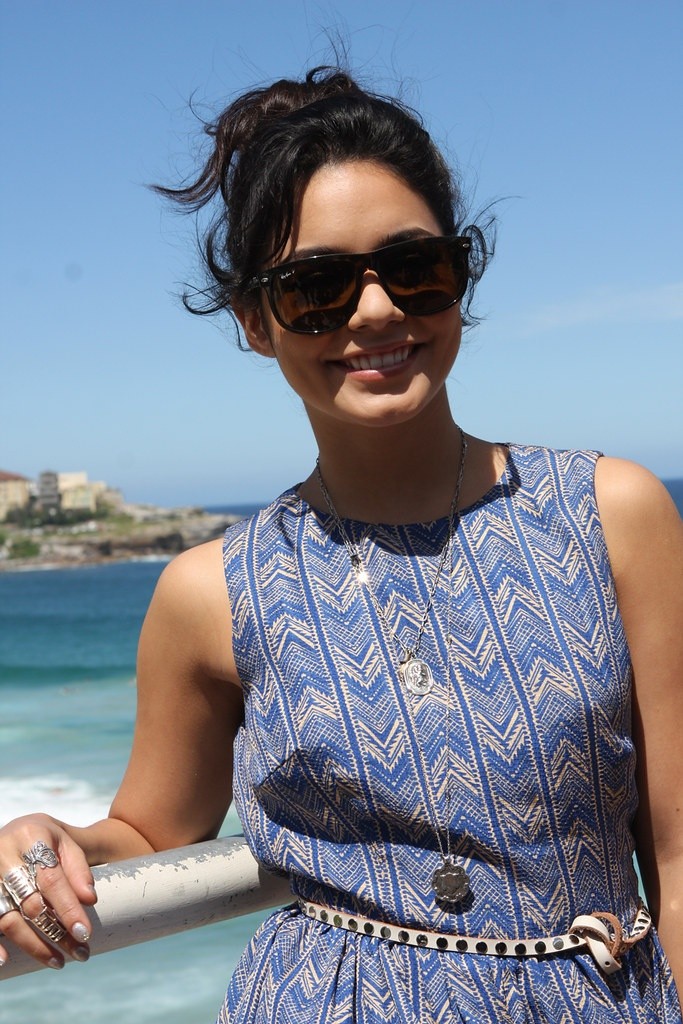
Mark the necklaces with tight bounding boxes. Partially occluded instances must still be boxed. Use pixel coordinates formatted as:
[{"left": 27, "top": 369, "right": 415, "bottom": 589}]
[{"left": 317, "top": 422, "right": 469, "bottom": 906}]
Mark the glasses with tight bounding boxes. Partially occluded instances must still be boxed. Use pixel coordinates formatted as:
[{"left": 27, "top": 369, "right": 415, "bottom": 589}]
[{"left": 249, "top": 236, "right": 472, "bottom": 335}]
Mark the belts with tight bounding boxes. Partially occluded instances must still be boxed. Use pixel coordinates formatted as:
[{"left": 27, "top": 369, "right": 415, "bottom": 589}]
[{"left": 296, "top": 895, "right": 652, "bottom": 974}]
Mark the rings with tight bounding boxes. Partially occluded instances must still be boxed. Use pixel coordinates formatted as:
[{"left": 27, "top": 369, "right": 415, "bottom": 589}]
[
  {"left": 2, "top": 866, "right": 67, "bottom": 942},
  {"left": 22, "top": 841, "right": 58, "bottom": 875},
  {"left": 0, "top": 883, "right": 19, "bottom": 917}
]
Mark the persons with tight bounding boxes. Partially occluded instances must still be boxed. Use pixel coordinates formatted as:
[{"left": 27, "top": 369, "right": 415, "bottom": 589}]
[{"left": 0, "top": 71, "right": 682, "bottom": 1023}]
